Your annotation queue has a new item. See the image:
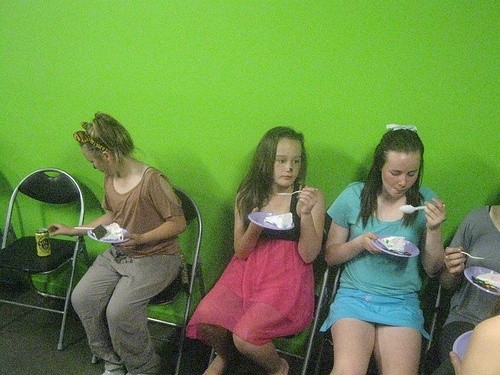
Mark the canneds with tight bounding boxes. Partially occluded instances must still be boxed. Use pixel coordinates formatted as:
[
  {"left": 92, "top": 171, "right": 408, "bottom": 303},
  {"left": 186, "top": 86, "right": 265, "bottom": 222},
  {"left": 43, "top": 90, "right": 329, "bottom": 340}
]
[{"left": 35, "top": 229, "right": 51, "bottom": 256}]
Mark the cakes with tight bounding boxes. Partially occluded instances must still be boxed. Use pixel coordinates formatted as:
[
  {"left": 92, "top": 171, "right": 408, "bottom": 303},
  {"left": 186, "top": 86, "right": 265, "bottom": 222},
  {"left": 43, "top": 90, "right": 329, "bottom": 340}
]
[
  {"left": 377, "top": 235, "right": 405, "bottom": 254},
  {"left": 91, "top": 222, "right": 128, "bottom": 241},
  {"left": 265, "top": 212, "right": 292, "bottom": 229},
  {"left": 475, "top": 271, "right": 500, "bottom": 289}
]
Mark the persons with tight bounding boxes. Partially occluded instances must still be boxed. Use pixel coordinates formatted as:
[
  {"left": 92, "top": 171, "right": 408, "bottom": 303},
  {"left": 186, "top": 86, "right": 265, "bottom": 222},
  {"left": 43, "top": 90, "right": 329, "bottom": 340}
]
[
  {"left": 186, "top": 126, "right": 325, "bottom": 375},
  {"left": 47, "top": 114, "right": 186, "bottom": 375},
  {"left": 434, "top": 205, "right": 500, "bottom": 375},
  {"left": 321, "top": 124, "right": 447, "bottom": 375}
]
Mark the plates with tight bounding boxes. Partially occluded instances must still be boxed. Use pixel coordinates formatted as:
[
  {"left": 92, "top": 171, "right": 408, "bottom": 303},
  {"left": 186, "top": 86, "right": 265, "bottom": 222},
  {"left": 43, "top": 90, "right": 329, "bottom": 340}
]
[
  {"left": 248, "top": 211, "right": 295, "bottom": 231},
  {"left": 87, "top": 227, "right": 131, "bottom": 244},
  {"left": 464, "top": 266, "right": 500, "bottom": 296},
  {"left": 372, "top": 235, "right": 420, "bottom": 257}
]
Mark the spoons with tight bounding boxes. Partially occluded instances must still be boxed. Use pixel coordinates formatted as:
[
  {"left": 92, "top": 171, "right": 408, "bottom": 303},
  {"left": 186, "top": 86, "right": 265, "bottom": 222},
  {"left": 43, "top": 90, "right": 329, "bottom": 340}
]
[
  {"left": 461, "top": 251, "right": 485, "bottom": 260},
  {"left": 277, "top": 190, "right": 301, "bottom": 196},
  {"left": 400, "top": 205, "right": 427, "bottom": 213}
]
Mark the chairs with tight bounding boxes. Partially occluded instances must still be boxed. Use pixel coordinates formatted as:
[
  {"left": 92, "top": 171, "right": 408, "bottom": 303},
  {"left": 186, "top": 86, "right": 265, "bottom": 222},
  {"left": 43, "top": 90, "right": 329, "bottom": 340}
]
[
  {"left": 147, "top": 185, "right": 202, "bottom": 375},
  {"left": 276, "top": 227, "right": 328, "bottom": 375},
  {"left": 0, "top": 168, "right": 84, "bottom": 350},
  {"left": 331, "top": 266, "right": 442, "bottom": 351}
]
